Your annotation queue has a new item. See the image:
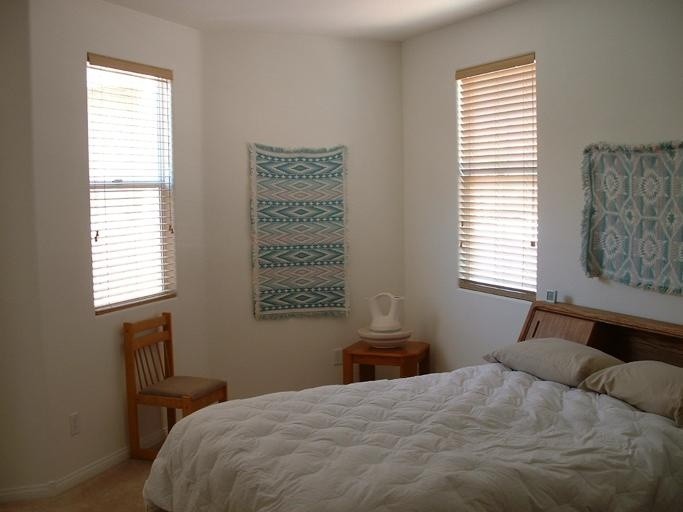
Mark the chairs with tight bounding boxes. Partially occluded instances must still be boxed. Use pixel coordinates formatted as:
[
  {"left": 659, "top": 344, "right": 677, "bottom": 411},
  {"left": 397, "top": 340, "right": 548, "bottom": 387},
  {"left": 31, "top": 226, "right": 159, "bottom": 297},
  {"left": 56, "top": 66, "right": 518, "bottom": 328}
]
[{"left": 123, "top": 313, "right": 228, "bottom": 460}]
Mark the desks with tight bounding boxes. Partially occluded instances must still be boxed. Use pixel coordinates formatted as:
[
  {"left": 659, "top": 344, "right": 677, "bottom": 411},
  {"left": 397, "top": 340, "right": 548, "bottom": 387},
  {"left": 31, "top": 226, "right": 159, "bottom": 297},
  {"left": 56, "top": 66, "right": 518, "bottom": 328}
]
[{"left": 343, "top": 341, "right": 429, "bottom": 385}]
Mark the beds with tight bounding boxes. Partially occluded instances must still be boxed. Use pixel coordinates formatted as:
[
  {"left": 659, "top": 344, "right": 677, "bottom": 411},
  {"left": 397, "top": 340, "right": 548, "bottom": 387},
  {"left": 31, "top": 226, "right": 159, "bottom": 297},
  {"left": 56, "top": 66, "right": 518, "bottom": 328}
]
[{"left": 141, "top": 299, "right": 683, "bottom": 511}]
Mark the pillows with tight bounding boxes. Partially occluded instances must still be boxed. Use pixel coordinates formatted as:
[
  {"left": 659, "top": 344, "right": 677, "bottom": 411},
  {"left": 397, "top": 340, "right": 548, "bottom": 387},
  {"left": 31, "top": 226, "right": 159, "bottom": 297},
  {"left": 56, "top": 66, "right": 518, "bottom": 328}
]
[
  {"left": 484, "top": 338, "right": 628, "bottom": 389},
  {"left": 581, "top": 359, "right": 683, "bottom": 429}
]
